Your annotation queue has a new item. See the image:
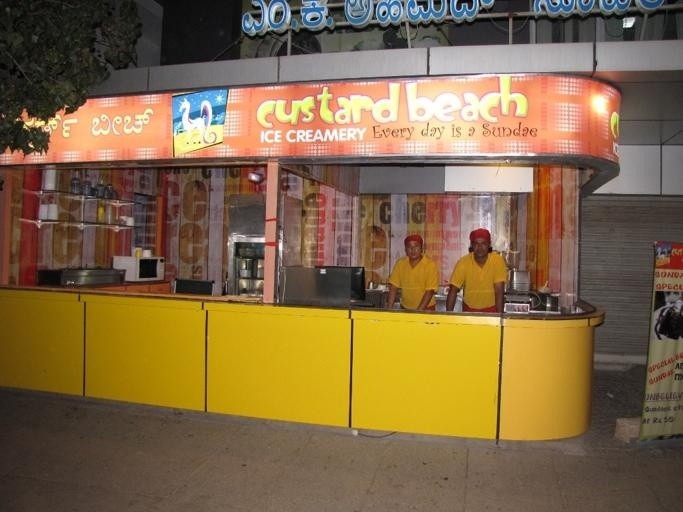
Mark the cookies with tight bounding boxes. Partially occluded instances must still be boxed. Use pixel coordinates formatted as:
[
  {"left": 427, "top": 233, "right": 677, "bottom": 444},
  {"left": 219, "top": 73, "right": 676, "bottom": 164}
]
[{"left": 112, "top": 255, "right": 167, "bottom": 283}]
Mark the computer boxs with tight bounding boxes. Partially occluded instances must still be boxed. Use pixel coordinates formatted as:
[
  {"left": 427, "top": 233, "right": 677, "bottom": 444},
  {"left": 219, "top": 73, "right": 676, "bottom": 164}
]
[{"left": 247, "top": 172, "right": 265, "bottom": 184}]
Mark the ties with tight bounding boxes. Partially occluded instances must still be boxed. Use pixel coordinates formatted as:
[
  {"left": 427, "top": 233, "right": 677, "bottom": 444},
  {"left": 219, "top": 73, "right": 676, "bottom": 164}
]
[{"left": 314, "top": 266, "right": 366, "bottom": 308}]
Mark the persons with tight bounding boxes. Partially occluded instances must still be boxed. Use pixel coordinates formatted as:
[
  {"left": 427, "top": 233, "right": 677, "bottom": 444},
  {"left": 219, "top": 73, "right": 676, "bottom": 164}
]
[
  {"left": 446, "top": 228, "right": 508, "bottom": 312},
  {"left": 387, "top": 236, "right": 439, "bottom": 310}
]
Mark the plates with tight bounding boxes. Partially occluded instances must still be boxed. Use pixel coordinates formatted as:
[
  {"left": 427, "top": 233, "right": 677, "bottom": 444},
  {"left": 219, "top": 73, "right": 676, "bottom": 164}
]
[
  {"left": 226, "top": 235, "right": 267, "bottom": 298},
  {"left": 97, "top": 279, "right": 171, "bottom": 294}
]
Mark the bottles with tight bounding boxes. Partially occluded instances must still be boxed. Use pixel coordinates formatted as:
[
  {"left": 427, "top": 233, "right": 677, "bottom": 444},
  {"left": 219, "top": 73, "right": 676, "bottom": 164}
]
[
  {"left": 405, "top": 235, "right": 423, "bottom": 247},
  {"left": 470, "top": 229, "right": 491, "bottom": 242}
]
